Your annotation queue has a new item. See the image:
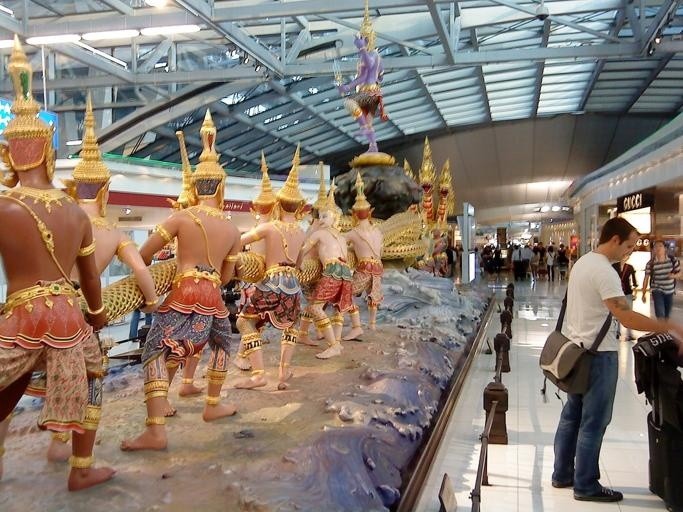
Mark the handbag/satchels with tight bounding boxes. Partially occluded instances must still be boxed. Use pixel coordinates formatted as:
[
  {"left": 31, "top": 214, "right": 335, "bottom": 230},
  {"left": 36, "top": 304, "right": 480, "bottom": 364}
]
[{"left": 538, "top": 330, "right": 594, "bottom": 395}]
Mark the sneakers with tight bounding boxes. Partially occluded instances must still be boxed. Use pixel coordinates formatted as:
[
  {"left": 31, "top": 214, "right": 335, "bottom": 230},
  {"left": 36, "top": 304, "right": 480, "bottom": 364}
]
[
  {"left": 574, "top": 486, "right": 623, "bottom": 502},
  {"left": 552, "top": 473, "right": 573, "bottom": 488}
]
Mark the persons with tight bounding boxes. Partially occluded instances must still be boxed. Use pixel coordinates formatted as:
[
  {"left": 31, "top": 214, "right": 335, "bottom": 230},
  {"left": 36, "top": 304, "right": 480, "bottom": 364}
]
[
  {"left": 550, "top": 218, "right": 682, "bottom": 503},
  {"left": 483, "top": 241, "right": 681, "bottom": 321},
  {"left": 341, "top": 26, "right": 385, "bottom": 152},
  {"left": 0, "top": 117, "right": 457, "bottom": 492}
]
